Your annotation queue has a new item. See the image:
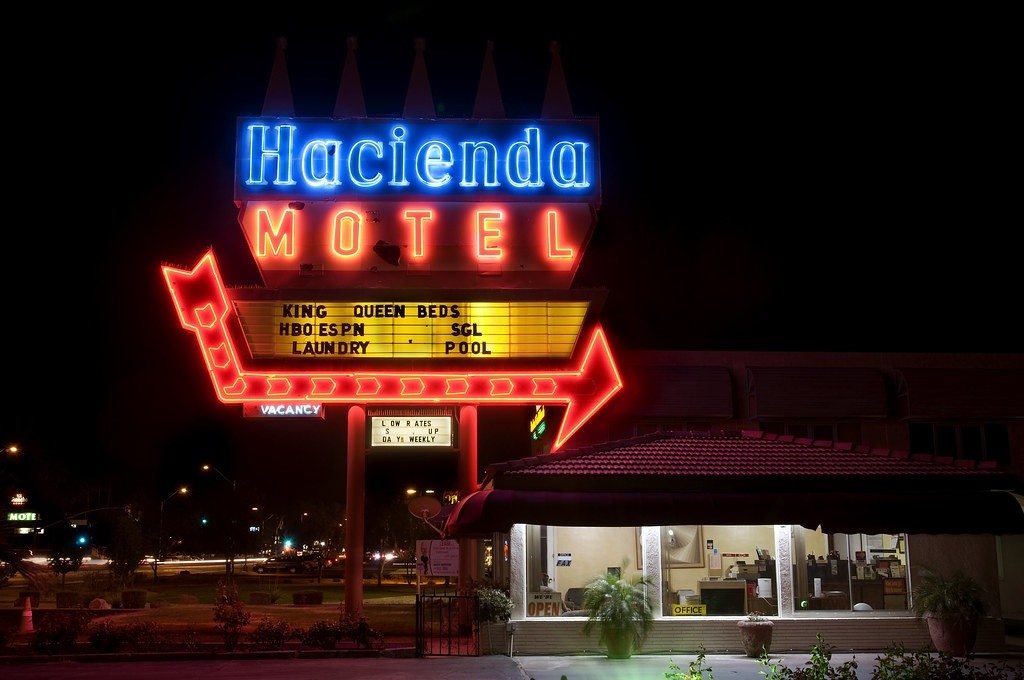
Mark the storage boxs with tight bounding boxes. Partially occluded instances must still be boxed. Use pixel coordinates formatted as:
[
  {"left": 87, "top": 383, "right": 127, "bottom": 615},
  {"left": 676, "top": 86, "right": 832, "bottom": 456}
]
[{"left": 736, "top": 559, "right": 765, "bottom": 580}]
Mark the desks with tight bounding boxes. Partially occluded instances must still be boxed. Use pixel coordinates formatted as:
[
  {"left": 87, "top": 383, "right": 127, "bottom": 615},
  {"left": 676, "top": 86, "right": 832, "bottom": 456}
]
[{"left": 808, "top": 578, "right": 883, "bottom": 603}]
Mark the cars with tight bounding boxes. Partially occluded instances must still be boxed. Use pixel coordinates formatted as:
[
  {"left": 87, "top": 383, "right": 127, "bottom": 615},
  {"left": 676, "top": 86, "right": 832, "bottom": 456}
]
[
  {"left": 304, "top": 554, "right": 333, "bottom": 573},
  {"left": 252, "top": 555, "right": 303, "bottom": 573}
]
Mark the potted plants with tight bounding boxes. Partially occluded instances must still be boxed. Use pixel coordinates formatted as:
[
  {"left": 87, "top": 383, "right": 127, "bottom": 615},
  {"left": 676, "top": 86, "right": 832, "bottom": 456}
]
[
  {"left": 472, "top": 586, "right": 512, "bottom": 655},
  {"left": 909, "top": 568, "right": 990, "bottom": 659},
  {"left": 738, "top": 610, "right": 774, "bottom": 658},
  {"left": 577, "top": 557, "right": 659, "bottom": 659}
]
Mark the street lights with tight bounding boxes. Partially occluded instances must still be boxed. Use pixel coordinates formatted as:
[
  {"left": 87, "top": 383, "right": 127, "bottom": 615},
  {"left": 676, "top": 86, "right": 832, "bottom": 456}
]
[
  {"left": 159, "top": 487, "right": 188, "bottom": 550},
  {"left": 203, "top": 465, "right": 236, "bottom": 491},
  {"left": 0, "top": 446, "right": 18, "bottom": 453}
]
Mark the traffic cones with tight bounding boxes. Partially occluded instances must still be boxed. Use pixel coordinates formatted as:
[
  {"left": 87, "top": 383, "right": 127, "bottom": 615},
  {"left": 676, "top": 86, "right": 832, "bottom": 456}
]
[{"left": 16, "top": 596, "right": 37, "bottom": 635}]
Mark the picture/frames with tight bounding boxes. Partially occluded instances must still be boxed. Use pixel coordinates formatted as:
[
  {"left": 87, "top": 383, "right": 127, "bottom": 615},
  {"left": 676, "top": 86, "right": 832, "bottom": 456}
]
[{"left": 635, "top": 525, "right": 706, "bottom": 570}]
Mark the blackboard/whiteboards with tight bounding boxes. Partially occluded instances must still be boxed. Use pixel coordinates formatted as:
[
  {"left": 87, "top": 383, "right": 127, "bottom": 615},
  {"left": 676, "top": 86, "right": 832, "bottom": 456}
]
[{"left": 699, "top": 587, "right": 746, "bottom": 615}]
